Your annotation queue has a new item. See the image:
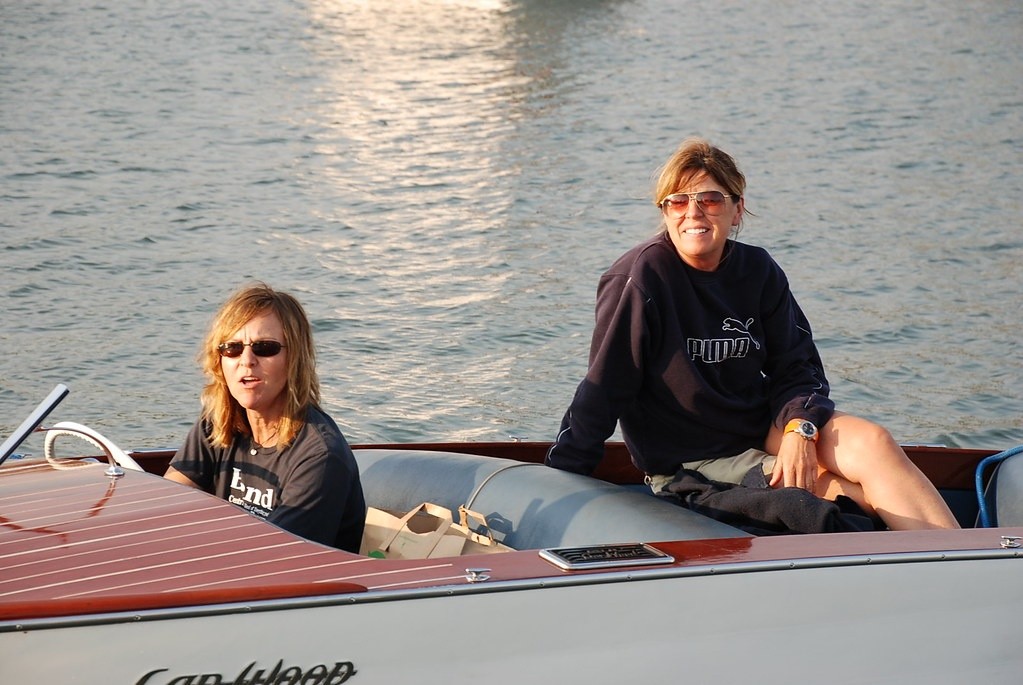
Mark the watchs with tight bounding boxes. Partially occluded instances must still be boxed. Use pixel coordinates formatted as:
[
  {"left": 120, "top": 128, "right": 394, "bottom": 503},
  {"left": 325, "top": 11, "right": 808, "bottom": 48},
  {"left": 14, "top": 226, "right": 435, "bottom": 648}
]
[{"left": 784, "top": 420, "right": 818, "bottom": 443}]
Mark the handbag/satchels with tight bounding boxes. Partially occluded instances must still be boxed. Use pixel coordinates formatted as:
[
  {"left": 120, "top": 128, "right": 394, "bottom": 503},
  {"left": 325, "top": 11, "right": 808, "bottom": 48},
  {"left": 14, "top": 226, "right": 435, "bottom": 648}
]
[{"left": 358, "top": 502, "right": 517, "bottom": 559}]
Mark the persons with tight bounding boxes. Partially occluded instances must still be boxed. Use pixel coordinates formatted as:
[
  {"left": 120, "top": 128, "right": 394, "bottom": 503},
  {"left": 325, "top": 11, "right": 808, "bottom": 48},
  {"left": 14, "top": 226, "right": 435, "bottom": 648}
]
[
  {"left": 163, "top": 286, "right": 366, "bottom": 554},
  {"left": 545, "top": 144, "right": 961, "bottom": 531}
]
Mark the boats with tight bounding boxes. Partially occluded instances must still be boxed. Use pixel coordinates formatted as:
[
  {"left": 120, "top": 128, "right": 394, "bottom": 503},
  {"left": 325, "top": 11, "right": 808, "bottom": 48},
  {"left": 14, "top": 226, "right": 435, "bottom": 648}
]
[{"left": 1, "top": 440, "right": 1023, "bottom": 685}]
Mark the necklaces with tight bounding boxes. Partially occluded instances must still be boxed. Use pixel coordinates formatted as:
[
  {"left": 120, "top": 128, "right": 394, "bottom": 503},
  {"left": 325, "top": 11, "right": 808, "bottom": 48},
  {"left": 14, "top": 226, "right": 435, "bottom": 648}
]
[{"left": 250, "top": 432, "right": 276, "bottom": 456}]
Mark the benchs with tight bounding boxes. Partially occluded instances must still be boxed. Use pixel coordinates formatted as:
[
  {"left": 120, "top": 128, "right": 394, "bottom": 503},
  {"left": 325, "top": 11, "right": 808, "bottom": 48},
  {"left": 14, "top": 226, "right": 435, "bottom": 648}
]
[{"left": 343, "top": 448, "right": 754, "bottom": 550}]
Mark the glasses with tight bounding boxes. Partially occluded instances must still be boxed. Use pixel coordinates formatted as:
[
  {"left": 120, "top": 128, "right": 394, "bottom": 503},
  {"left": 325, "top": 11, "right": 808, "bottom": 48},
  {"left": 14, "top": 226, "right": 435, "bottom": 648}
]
[
  {"left": 216, "top": 340, "right": 286, "bottom": 358},
  {"left": 659, "top": 191, "right": 733, "bottom": 220}
]
